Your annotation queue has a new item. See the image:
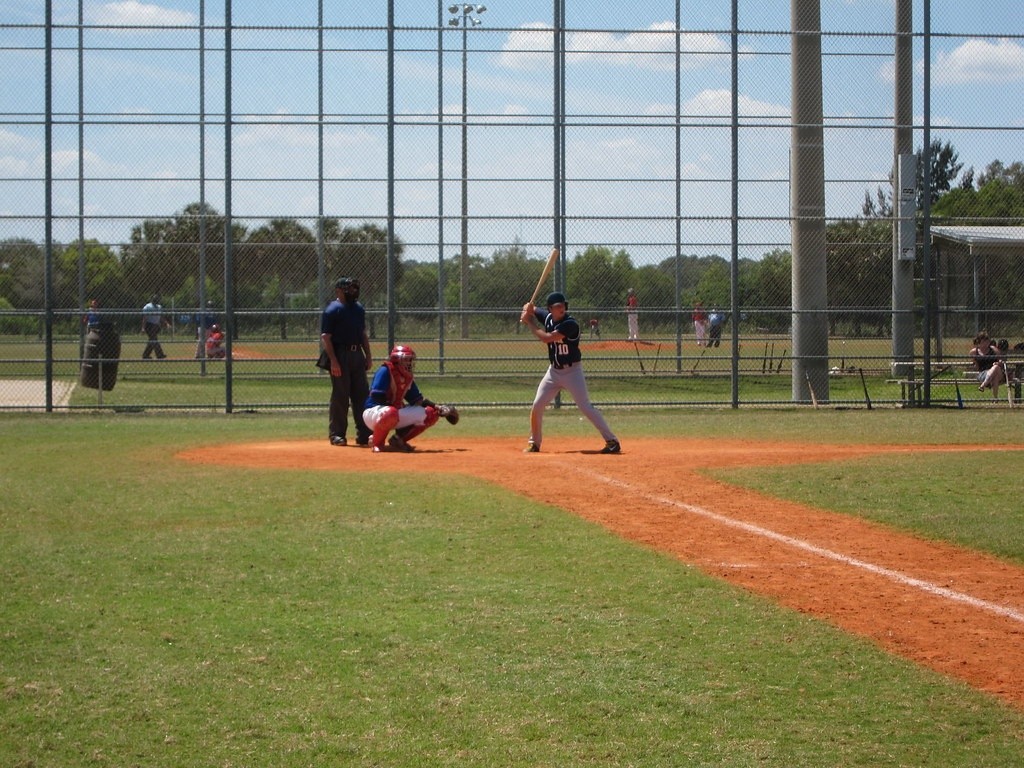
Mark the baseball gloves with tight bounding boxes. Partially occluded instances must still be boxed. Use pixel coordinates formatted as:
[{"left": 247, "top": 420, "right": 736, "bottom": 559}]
[{"left": 438, "top": 405, "right": 459, "bottom": 425}]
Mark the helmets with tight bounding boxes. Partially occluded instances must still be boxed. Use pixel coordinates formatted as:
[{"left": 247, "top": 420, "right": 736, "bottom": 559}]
[
  {"left": 546, "top": 292, "right": 569, "bottom": 313},
  {"left": 390, "top": 345, "right": 416, "bottom": 379},
  {"left": 207, "top": 301, "right": 214, "bottom": 307},
  {"left": 336, "top": 277, "right": 360, "bottom": 303},
  {"left": 211, "top": 324, "right": 220, "bottom": 332}
]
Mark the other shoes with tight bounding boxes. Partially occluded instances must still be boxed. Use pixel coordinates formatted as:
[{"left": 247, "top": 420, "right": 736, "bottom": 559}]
[
  {"left": 372, "top": 445, "right": 386, "bottom": 453},
  {"left": 359, "top": 440, "right": 367, "bottom": 446},
  {"left": 330, "top": 435, "right": 347, "bottom": 445},
  {"left": 600, "top": 440, "right": 620, "bottom": 453},
  {"left": 523, "top": 446, "right": 539, "bottom": 453},
  {"left": 389, "top": 433, "right": 413, "bottom": 452}
]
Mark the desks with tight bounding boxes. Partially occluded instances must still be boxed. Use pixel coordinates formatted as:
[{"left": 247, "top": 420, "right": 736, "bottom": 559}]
[{"left": 892, "top": 361, "right": 1024, "bottom": 408}]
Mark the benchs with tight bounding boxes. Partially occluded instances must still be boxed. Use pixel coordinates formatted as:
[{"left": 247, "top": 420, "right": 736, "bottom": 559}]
[{"left": 885, "top": 377, "right": 1024, "bottom": 408}]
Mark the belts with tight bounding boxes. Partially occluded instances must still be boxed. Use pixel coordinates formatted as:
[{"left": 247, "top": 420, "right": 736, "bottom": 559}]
[
  {"left": 335, "top": 345, "right": 363, "bottom": 352},
  {"left": 554, "top": 363, "right": 571, "bottom": 370}
]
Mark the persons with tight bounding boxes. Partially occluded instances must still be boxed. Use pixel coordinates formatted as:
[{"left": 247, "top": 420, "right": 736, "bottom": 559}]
[
  {"left": 195, "top": 302, "right": 217, "bottom": 358},
  {"left": 142, "top": 294, "right": 171, "bottom": 359},
  {"left": 521, "top": 292, "right": 620, "bottom": 453},
  {"left": 362, "top": 345, "right": 459, "bottom": 452},
  {"left": 83, "top": 301, "right": 99, "bottom": 334},
  {"left": 206, "top": 325, "right": 225, "bottom": 359},
  {"left": 626, "top": 289, "right": 639, "bottom": 339},
  {"left": 970, "top": 333, "right": 1005, "bottom": 403},
  {"left": 315, "top": 278, "right": 374, "bottom": 445},
  {"left": 692, "top": 303, "right": 725, "bottom": 347},
  {"left": 583, "top": 320, "right": 602, "bottom": 338}
]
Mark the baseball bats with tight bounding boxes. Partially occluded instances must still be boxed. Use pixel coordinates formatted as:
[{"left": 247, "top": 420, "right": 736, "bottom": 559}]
[
  {"left": 691, "top": 350, "right": 706, "bottom": 373},
  {"left": 770, "top": 343, "right": 775, "bottom": 368},
  {"left": 777, "top": 349, "right": 786, "bottom": 372},
  {"left": 806, "top": 372, "right": 819, "bottom": 410},
  {"left": 762, "top": 343, "right": 768, "bottom": 373},
  {"left": 952, "top": 365, "right": 963, "bottom": 408},
  {"left": 841, "top": 341, "right": 845, "bottom": 372},
  {"left": 653, "top": 343, "right": 661, "bottom": 374},
  {"left": 634, "top": 342, "right": 646, "bottom": 375},
  {"left": 859, "top": 368, "right": 871, "bottom": 410},
  {"left": 520, "top": 248, "right": 559, "bottom": 324},
  {"left": 1003, "top": 362, "right": 1013, "bottom": 409}
]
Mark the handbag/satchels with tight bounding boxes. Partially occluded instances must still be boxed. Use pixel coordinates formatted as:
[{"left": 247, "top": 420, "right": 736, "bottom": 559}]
[{"left": 316, "top": 350, "right": 329, "bottom": 369}]
